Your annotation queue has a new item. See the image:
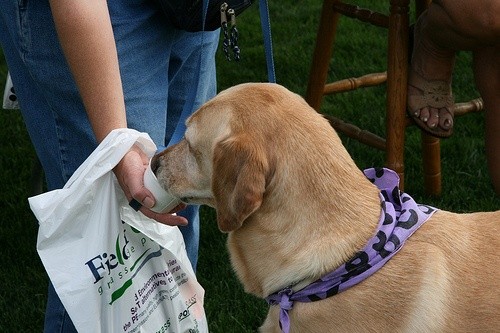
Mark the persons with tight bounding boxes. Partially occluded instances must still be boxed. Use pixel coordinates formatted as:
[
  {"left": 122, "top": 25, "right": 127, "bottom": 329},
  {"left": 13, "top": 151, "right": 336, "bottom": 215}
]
[
  {"left": 406, "top": 1, "right": 500, "bottom": 210},
  {"left": 0, "top": 1, "right": 222, "bottom": 333}
]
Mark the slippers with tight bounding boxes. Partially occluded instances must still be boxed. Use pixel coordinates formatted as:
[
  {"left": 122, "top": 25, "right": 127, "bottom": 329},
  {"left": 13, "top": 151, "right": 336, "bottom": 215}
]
[{"left": 403, "top": 21, "right": 456, "bottom": 138}]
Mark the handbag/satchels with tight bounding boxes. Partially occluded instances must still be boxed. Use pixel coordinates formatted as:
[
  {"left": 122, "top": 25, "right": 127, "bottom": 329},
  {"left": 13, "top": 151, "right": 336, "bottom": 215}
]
[
  {"left": 152, "top": 0, "right": 257, "bottom": 62},
  {"left": 28, "top": 129, "right": 210, "bottom": 333}
]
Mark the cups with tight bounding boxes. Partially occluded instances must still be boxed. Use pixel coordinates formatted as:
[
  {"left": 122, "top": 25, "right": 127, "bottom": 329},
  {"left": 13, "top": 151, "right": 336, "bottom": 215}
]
[{"left": 142, "top": 158, "right": 181, "bottom": 214}]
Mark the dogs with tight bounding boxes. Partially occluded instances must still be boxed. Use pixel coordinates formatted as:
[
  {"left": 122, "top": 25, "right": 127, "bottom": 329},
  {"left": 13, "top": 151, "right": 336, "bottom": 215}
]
[{"left": 149, "top": 82, "right": 500, "bottom": 333}]
